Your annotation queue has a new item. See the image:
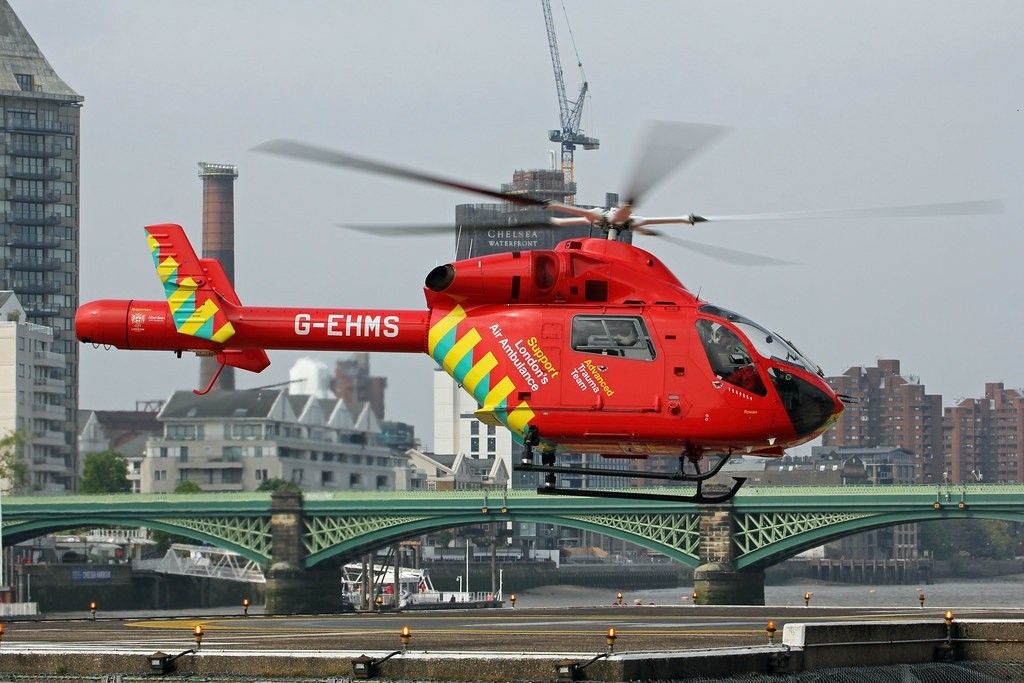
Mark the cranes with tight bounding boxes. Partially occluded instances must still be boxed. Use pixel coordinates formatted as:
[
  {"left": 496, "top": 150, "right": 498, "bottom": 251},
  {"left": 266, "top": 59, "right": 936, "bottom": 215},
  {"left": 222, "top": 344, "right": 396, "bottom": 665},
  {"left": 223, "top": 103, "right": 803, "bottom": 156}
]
[{"left": 540, "top": 0, "right": 601, "bottom": 207}]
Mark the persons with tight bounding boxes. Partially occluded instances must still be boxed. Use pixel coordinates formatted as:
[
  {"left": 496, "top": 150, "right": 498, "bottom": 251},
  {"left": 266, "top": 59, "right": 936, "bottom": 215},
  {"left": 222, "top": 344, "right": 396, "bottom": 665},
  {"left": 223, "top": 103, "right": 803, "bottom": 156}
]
[
  {"left": 708, "top": 326, "right": 745, "bottom": 370},
  {"left": 386, "top": 584, "right": 393, "bottom": 598}
]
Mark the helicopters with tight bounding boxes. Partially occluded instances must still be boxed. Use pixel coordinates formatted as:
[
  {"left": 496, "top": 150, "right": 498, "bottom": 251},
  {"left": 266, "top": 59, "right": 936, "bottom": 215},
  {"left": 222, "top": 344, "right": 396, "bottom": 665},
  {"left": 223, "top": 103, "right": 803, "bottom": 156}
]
[{"left": 70, "top": 121, "right": 1003, "bottom": 503}]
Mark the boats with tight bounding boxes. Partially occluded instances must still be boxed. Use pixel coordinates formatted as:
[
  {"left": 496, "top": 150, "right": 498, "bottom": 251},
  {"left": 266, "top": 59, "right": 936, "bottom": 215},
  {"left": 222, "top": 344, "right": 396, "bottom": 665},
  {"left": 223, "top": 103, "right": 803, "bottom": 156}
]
[{"left": 371, "top": 570, "right": 439, "bottom": 610}]
